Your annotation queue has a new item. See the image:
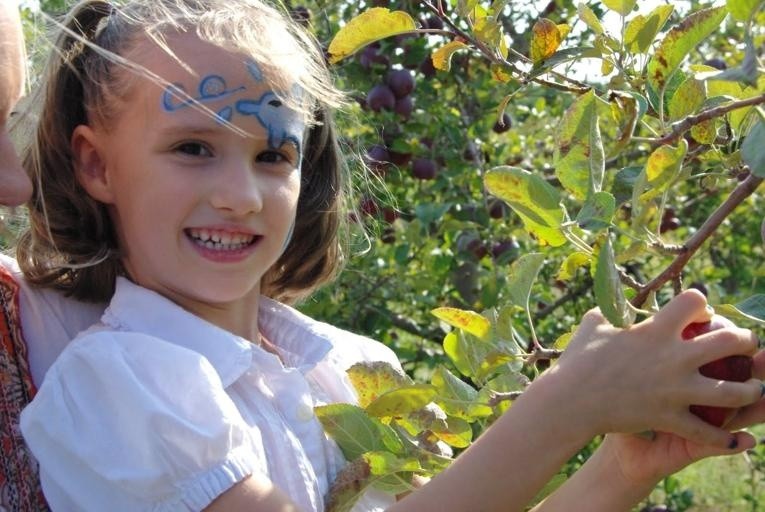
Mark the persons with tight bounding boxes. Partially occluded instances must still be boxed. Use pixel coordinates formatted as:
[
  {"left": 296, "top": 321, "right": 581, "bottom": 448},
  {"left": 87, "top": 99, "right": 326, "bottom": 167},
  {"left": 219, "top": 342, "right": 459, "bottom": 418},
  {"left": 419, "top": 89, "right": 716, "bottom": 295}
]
[
  {"left": 0, "top": 0, "right": 765, "bottom": 512},
  {"left": 0, "top": 0, "right": 110, "bottom": 512}
]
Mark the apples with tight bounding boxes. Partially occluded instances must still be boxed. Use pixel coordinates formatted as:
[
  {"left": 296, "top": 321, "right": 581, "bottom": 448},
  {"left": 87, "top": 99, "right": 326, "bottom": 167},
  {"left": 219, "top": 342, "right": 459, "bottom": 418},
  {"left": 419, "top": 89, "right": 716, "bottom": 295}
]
[
  {"left": 677, "top": 314, "right": 754, "bottom": 429},
  {"left": 660, "top": 204, "right": 682, "bottom": 233},
  {"left": 292, "top": 0, "right": 517, "bottom": 263},
  {"left": 705, "top": 59, "right": 725, "bottom": 71}
]
